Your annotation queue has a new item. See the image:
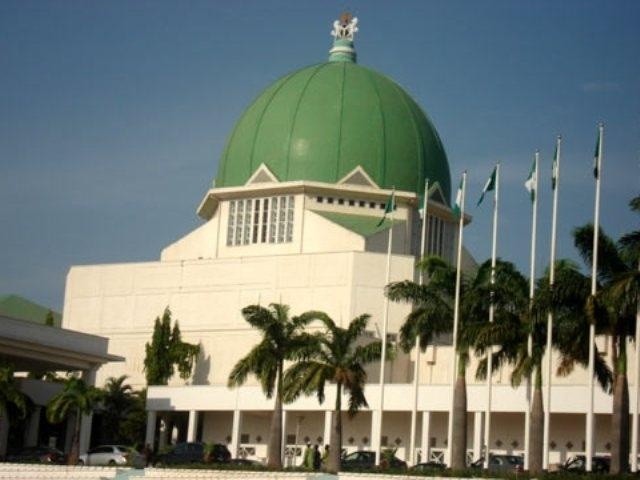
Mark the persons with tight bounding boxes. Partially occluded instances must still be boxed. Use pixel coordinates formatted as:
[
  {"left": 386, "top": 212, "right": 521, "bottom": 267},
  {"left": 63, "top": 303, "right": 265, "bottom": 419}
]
[{"left": 304, "top": 441, "right": 330, "bottom": 470}]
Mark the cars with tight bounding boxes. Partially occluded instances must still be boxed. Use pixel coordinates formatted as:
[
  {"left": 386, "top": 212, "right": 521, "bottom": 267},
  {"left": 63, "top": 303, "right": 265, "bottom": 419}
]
[
  {"left": 0, "top": 443, "right": 231, "bottom": 466},
  {"left": 341, "top": 451, "right": 523, "bottom": 474},
  {"left": 564, "top": 457, "right": 612, "bottom": 473}
]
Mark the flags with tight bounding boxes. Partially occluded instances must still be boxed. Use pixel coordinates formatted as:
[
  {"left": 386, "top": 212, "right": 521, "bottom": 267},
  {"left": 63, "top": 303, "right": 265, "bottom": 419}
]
[
  {"left": 375, "top": 193, "right": 393, "bottom": 227},
  {"left": 452, "top": 176, "right": 463, "bottom": 219},
  {"left": 524, "top": 156, "right": 536, "bottom": 201},
  {"left": 476, "top": 166, "right": 496, "bottom": 209},
  {"left": 593, "top": 127, "right": 600, "bottom": 178},
  {"left": 417, "top": 186, "right": 425, "bottom": 220},
  {"left": 551, "top": 144, "right": 558, "bottom": 190}
]
